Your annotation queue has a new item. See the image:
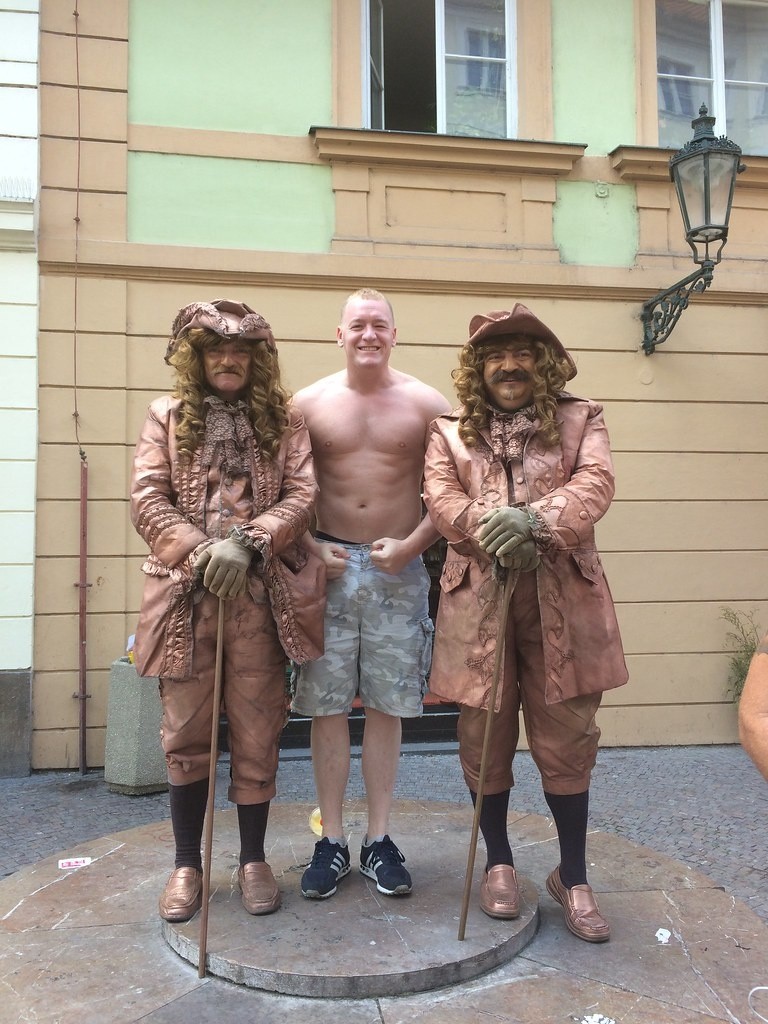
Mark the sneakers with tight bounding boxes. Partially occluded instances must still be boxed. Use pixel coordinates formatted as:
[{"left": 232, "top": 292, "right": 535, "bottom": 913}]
[
  {"left": 359, "top": 833, "right": 413, "bottom": 896},
  {"left": 300, "top": 837, "right": 352, "bottom": 899}
]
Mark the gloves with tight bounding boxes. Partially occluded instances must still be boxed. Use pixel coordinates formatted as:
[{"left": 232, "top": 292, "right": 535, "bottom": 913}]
[
  {"left": 477, "top": 506, "right": 536, "bottom": 557},
  {"left": 498, "top": 540, "right": 541, "bottom": 573},
  {"left": 192, "top": 537, "right": 255, "bottom": 600}
]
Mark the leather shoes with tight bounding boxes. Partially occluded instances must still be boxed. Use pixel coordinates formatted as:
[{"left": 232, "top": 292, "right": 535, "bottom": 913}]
[
  {"left": 238, "top": 862, "right": 282, "bottom": 916},
  {"left": 479, "top": 861, "right": 520, "bottom": 919},
  {"left": 545, "top": 864, "right": 611, "bottom": 943},
  {"left": 159, "top": 867, "right": 203, "bottom": 923}
]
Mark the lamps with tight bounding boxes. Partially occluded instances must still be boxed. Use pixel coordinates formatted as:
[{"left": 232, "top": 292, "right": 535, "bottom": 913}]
[{"left": 642, "top": 99, "right": 748, "bottom": 357}]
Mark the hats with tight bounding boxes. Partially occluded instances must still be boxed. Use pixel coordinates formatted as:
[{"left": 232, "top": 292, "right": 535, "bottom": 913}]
[
  {"left": 164, "top": 299, "right": 277, "bottom": 366},
  {"left": 467, "top": 303, "right": 577, "bottom": 381}
]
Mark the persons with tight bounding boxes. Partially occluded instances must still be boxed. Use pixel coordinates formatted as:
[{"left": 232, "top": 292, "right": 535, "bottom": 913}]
[
  {"left": 738, "top": 631, "right": 768, "bottom": 780},
  {"left": 130, "top": 298, "right": 327, "bottom": 922},
  {"left": 285, "top": 288, "right": 455, "bottom": 899},
  {"left": 424, "top": 302, "right": 629, "bottom": 943}
]
